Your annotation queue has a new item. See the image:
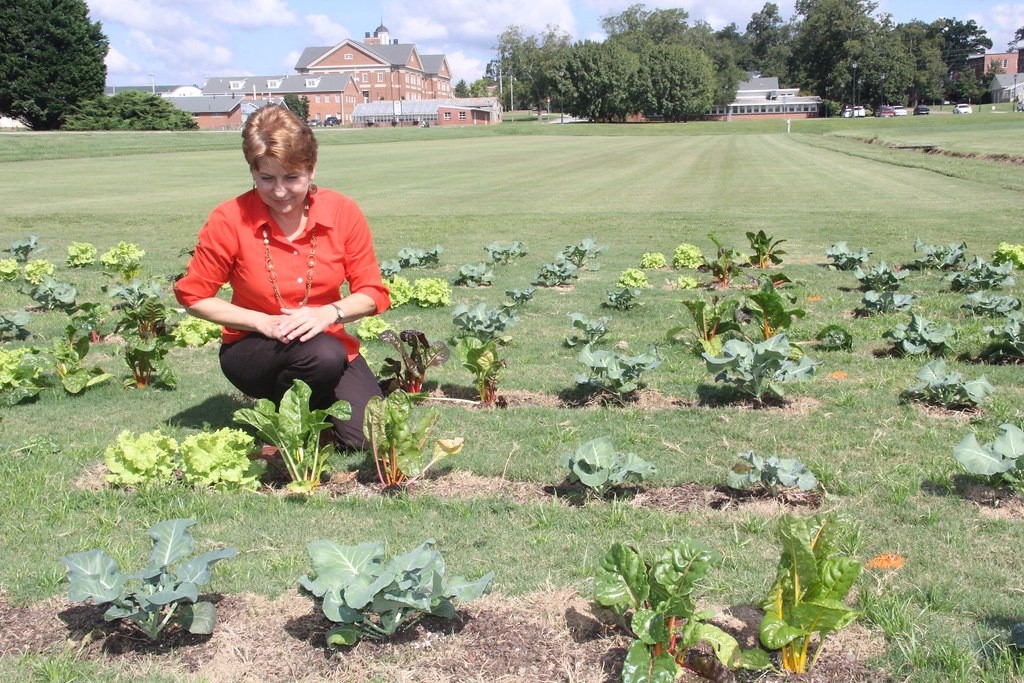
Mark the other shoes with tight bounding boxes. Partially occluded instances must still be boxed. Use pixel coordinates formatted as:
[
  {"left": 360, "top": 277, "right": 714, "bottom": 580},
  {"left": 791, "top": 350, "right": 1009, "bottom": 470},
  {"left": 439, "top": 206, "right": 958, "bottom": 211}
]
[{"left": 262, "top": 439, "right": 314, "bottom": 481}]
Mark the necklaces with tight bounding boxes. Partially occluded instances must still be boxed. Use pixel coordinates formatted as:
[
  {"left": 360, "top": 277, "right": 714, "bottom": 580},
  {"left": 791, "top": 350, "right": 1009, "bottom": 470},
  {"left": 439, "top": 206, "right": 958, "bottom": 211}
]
[{"left": 260, "top": 224, "right": 319, "bottom": 309}]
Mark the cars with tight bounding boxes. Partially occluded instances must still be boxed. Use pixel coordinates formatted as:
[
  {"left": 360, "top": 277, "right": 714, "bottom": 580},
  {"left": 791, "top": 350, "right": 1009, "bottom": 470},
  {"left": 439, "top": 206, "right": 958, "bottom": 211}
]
[
  {"left": 953, "top": 104, "right": 972, "bottom": 114},
  {"left": 913, "top": 104, "right": 930, "bottom": 114},
  {"left": 849, "top": 105, "right": 866, "bottom": 118},
  {"left": 308, "top": 119, "right": 322, "bottom": 127},
  {"left": 841, "top": 109, "right": 852, "bottom": 118},
  {"left": 875, "top": 106, "right": 894, "bottom": 117},
  {"left": 892, "top": 106, "right": 907, "bottom": 116},
  {"left": 323, "top": 117, "right": 340, "bottom": 126}
]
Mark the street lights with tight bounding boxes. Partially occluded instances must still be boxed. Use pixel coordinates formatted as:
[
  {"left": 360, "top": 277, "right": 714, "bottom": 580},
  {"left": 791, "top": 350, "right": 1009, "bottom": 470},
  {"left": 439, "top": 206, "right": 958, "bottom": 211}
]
[
  {"left": 559, "top": 69, "right": 564, "bottom": 124},
  {"left": 978, "top": 79, "right": 983, "bottom": 113},
  {"left": 825, "top": 87, "right": 828, "bottom": 118},
  {"left": 852, "top": 61, "right": 857, "bottom": 119},
  {"left": 881, "top": 73, "right": 885, "bottom": 106},
  {"left": 939, "top": 78, "right": 944, "bottom": 111},
  {"left": 1013, "top": 75, "right": 1018, "bottom": 113},
  {"left": 858, "top": 78, "right": 861, "bottom": 117}
]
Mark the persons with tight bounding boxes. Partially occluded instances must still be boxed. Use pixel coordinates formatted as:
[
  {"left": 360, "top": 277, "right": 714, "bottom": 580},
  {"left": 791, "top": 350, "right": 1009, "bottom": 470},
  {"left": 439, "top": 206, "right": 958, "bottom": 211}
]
[{"left": 173, "top": 103, "right": 393, "bottom": 451}]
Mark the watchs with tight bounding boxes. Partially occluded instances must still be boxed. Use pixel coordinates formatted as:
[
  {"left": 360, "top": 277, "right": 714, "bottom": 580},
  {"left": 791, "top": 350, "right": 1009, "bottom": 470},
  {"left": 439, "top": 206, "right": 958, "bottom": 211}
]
[{"left": 329, "top": 303, "right": 345, "bottom": 324}]
